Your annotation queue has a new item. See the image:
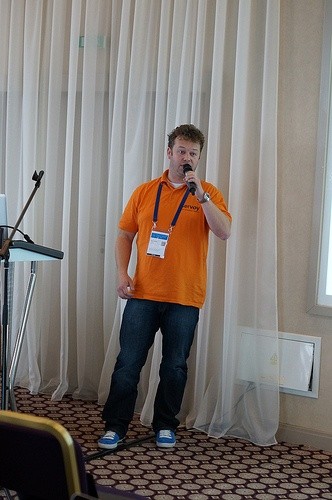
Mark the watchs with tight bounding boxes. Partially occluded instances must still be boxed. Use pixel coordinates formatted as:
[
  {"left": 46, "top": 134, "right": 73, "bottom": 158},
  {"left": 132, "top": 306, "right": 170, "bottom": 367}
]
[{"left": 196, "top": 192, "right": 210, "bottom": 204}]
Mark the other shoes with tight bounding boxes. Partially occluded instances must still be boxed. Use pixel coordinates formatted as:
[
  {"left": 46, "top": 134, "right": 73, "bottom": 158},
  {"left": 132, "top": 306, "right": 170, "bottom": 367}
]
[
  {"left": 98, "top": 431, "right": 125, "bottom": 449},
  {"left": 156, "top": 430, "right": 176, "bottom": 447}
]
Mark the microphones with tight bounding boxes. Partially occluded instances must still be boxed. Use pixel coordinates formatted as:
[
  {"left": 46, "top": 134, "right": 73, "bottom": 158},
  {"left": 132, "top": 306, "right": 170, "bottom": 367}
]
[
  {"left": 183, "top": 164, "right": 196, "bottom": 196},
  {"left": 0, "top": 225, "right": 34, "bottom": 244}
]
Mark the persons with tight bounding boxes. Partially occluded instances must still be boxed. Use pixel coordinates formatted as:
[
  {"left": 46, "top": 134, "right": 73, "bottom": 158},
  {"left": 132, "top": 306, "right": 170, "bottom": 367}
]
[{"left": 97, "top": 123, "right": 232, "bottom": 449}]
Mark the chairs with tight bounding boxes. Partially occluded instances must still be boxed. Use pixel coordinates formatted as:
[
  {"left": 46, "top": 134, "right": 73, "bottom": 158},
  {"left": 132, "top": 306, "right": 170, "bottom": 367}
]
[{"left": 0, "top": 408, "right": 146, "bottom": 500}]
